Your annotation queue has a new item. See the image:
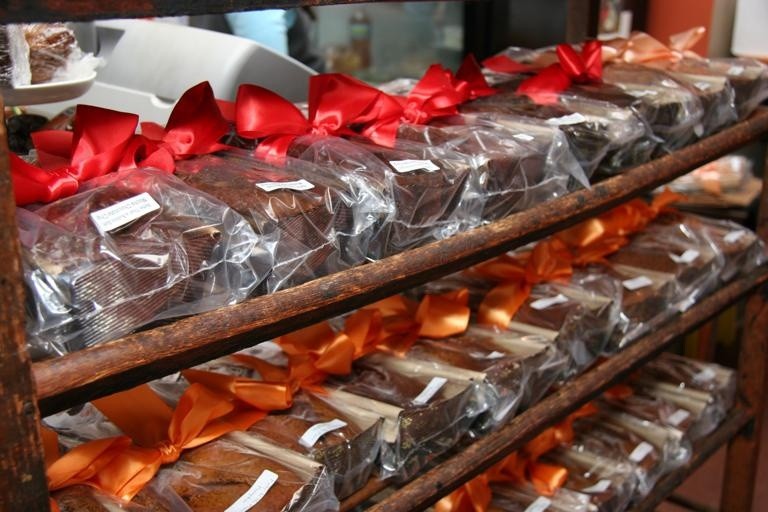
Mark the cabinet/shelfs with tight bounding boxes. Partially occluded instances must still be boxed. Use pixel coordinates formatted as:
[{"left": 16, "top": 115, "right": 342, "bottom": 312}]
[{"left": 0, "top": 89, "right": 768, "bottom": 512}]
